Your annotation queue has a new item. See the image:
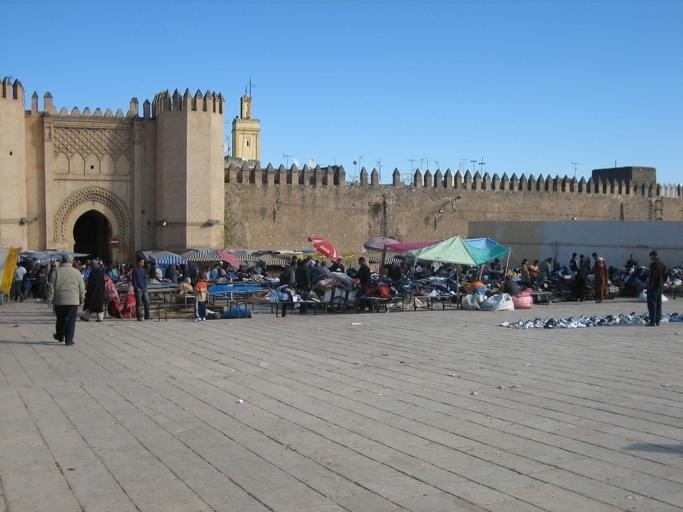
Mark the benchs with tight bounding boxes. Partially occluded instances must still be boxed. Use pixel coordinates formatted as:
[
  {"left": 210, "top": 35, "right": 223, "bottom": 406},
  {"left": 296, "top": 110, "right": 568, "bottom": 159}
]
[
  {"left": 532, "top": 291, "right": 552, "bottom": 304},
  {"left": 360, "top": 295, "right": 452, "bottom": 312},
  {"left": 212, "top": 300, "right": 256, "bottom": 318},
  {"left": 276, "top": 301, "right": 332, "bottom": 317}
]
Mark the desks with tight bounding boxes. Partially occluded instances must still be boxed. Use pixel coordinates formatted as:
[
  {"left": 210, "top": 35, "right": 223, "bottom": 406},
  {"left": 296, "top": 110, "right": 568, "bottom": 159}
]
[
  {"left": 104, "top": 286, "right": 182, "bottom": 322},
  {"left": 203, "top": 290, "right": 267, "bottom": 319}
]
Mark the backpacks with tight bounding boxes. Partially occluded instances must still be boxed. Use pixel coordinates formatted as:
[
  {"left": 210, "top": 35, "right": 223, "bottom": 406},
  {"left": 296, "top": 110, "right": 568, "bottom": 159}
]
[
  {"left": 461, "top": 291, "right": 487, "bottom": 310},
  {"left": 480, "top": 287, "right": 534, "bottom": 312}
]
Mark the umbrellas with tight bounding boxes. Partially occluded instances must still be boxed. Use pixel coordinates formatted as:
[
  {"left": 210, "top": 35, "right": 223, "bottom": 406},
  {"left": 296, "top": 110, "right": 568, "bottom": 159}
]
[
  {"left": 362, "top": 236, "right": 401, "bottom": 252},
  {"left": 306, "top": 235, "right": 341, "bottom": 263}
]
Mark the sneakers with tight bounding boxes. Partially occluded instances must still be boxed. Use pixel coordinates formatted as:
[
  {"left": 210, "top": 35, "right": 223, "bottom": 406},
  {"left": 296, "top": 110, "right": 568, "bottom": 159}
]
[
  {"left": 194, "top": 317, "right": 207, "bottom": 322},
  {"left": 497, "top": 311, "right": 682, "bottom": 328},
  {"left": 52, "top": 333, "right": 74, "bottom": 347},
  {"left": 79, "top": 312, "right": 153, "bottom": 323}
]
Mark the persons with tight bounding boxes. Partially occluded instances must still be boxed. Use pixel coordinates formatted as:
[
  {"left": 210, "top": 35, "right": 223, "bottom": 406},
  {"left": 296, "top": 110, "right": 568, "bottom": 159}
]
[
  {"left": 45, "top": 254, "right": 86, "bottom": 346},
  {"left": 277, "top": 256, "right": 371, "bottom": 300},
  {"left": 10, "top": 256, "right": 106, "bottom": 322},
  {"left": 164, "top": 260, "right": 268, "bottom": 284},
  {"left": 193, "top": 275, "right": 208, "bottom": 322},
  {"left": 386, "top": 250, "right": 591, "bottom": 302},
  {"left": 130, "top": 257, "right": 152, "bottom": 322},
  {"left": 624, "top": 254, "right": 638, "bottom": 268},
  {"left": 586, "top": 251, "right": 609, "bottom": 304},
  {"left": 644, "top": 250, "right": 669, "bottom": 327},
  {"left": 179, "top": 277, "right": 193, "bottom": 294}
]
